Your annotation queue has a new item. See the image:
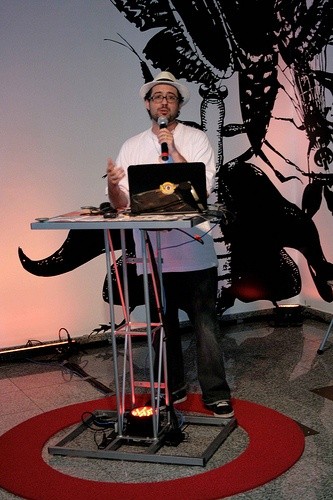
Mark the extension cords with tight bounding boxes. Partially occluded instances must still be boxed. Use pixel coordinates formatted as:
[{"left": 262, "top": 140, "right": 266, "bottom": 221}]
[{"left": 97, "top": 430, "right": 119, "bottom": 449}]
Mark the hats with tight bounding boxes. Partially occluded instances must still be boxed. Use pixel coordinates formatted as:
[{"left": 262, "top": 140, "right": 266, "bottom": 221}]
[{"left": 140, "top": 71, "right": 190, "bottom": 108}]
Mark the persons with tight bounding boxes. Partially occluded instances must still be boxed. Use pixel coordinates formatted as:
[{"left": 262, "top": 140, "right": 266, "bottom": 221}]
[{"left": 106, "top": 71, "right": 236, "bottom": 418}]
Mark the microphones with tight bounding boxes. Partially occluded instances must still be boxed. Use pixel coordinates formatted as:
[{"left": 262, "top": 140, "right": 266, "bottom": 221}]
[{"left": 157, "top": 116, "right": 169, "bottom": 161}]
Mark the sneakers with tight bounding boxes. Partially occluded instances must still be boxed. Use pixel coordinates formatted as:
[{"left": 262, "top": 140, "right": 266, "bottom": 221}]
[
  {"left": 206, "top": 400, "right": 234, "bottom": 418},
  {"left": 145, "top": 388, "right": 188, "bottom": 411}
]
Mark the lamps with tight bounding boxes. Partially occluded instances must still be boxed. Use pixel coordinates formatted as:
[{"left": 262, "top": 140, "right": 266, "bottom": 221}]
[{"left": 124, "top": 402, "right": 163, "bottom": 441}]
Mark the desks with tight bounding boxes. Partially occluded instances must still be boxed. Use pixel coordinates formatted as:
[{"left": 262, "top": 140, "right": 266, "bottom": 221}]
[{"left": 28, "top": 208, "right": 239, "bottom": 468}]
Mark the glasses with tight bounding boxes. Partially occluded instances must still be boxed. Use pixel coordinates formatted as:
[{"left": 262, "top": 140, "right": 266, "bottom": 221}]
[{"left": 148, "top": 94, "right": 179, "bottom": 104}]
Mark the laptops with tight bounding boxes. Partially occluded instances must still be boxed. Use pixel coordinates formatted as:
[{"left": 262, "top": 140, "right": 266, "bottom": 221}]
[{"left": 127, "top": 161, "right": 208, "bottom": 215}]
